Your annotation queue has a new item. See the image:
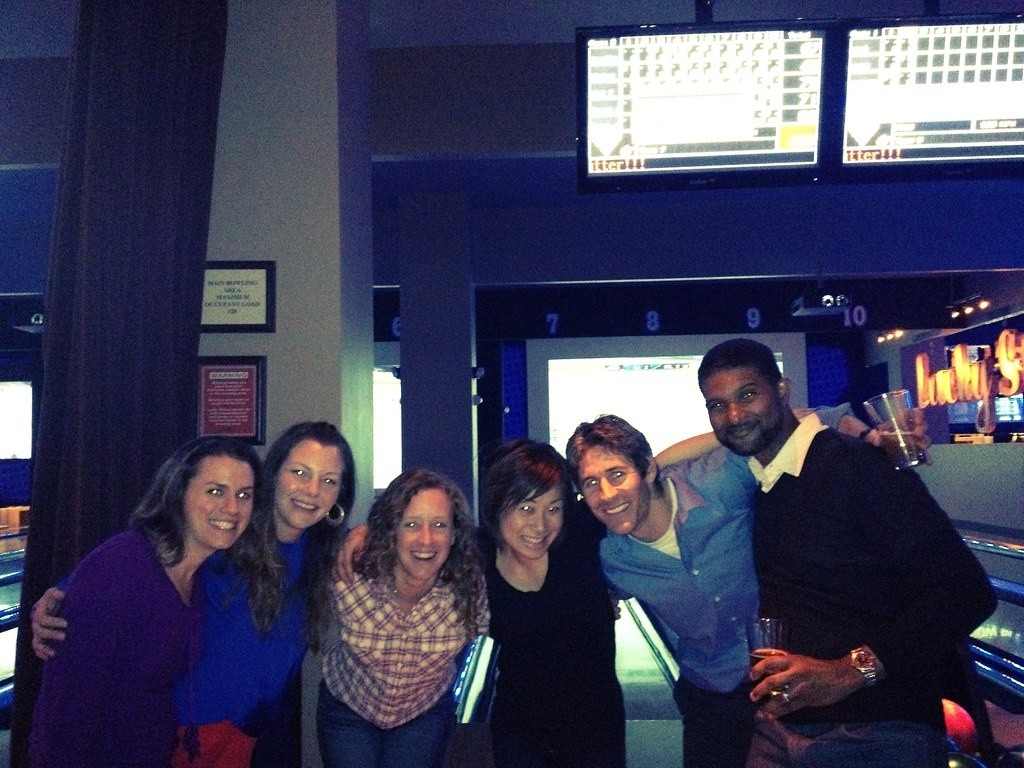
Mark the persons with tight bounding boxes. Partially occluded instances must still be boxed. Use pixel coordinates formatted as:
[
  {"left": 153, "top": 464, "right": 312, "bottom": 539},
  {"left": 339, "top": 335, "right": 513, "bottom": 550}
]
[
  {"left": 309, "top": 466, "right": 621, "bottom": 768},
  {"left": 330, "top": 407, "right": 820, "bottom": 768},
  {"left": 29, "top": 435, "right": 262, "bottom": 768},
  {"left": 31, "top": 421, "right": 356, "bottom": 768},
  {"left": 566, "top": 401, "right": 933, "bottom": 768},
  {"left": 697, "top": 339, "right": 999, "bottom": 768}
]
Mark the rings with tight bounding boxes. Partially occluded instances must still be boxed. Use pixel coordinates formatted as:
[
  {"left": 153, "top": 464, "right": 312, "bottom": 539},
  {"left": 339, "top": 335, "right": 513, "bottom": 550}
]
[{"left": 783, "top": 692, "right": 790, "bottom": 703}]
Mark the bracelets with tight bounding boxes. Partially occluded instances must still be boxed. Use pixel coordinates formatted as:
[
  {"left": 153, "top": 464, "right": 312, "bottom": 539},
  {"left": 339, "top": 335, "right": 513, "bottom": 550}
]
[{"left": 860, "top": 428, "right": 873, "bottom": 438}]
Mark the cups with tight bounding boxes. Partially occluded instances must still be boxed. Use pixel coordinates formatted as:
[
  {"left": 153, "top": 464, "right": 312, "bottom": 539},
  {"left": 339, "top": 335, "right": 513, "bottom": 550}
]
[
  {"left": 744, "top": 617, "right": 785, "bottom": 705},
  {"left": 863, "top": 389, "right": 927, "bottom": 469}
]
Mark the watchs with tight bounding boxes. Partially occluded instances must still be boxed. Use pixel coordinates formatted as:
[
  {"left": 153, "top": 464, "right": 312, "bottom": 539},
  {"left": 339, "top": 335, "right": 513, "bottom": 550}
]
[{"left": 851, "top": 648, "right": 876, "bottom": 687}]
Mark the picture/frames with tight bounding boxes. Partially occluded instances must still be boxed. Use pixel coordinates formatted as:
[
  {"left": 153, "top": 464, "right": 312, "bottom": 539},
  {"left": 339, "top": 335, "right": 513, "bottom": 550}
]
[
  {"left": 197, "top": 356, "right": 267, "bottom": 445},
  {"left": 196, "top": 260, "right": 276, "bottom": 332}
]
